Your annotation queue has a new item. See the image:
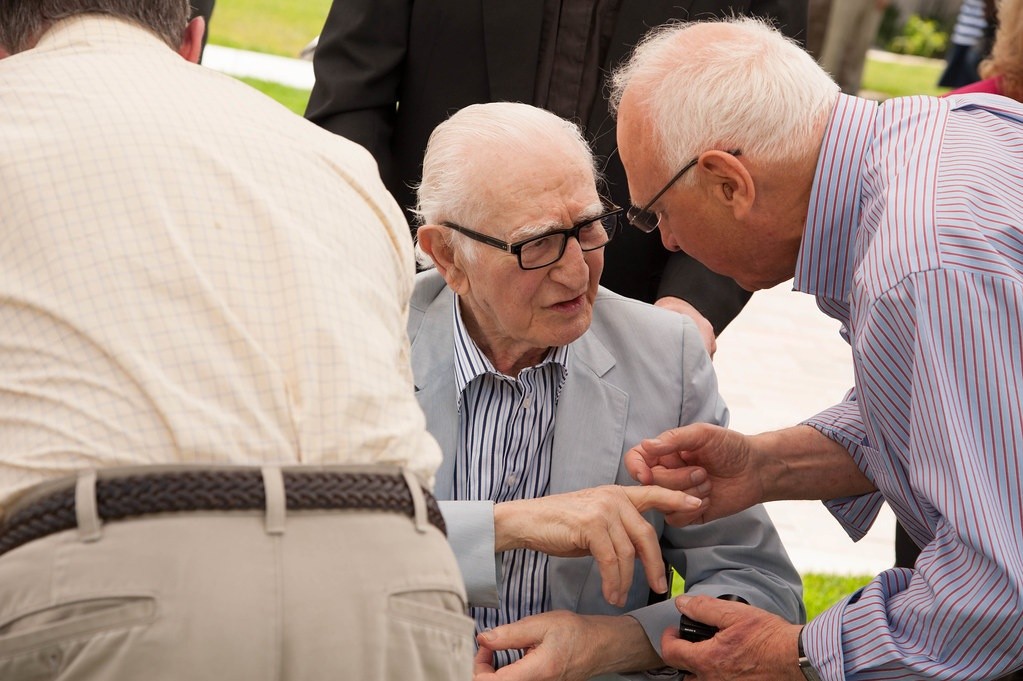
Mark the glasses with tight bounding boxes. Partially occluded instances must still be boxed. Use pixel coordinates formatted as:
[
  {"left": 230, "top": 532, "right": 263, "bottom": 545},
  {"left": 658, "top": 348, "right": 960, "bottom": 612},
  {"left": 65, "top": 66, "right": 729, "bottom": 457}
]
[
  {"left": 626, "top": 148, "right": 743, "bottom": 233},
  {"left": 437, "top": 190, "right": 625, "bottom": 270}
]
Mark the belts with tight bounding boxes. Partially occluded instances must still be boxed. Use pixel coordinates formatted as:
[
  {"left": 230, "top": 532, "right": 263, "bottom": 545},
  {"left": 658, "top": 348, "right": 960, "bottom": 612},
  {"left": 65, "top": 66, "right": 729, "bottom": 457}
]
[{"left": 0, "top": 468, "right": 448, "bottom": 557}]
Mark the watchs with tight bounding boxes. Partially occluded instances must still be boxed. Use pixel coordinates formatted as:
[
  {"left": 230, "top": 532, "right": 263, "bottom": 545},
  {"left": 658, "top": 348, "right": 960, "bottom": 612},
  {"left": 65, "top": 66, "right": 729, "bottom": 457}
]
[{"left": 798, "top": 625, "right": 821, "bottom": 681}]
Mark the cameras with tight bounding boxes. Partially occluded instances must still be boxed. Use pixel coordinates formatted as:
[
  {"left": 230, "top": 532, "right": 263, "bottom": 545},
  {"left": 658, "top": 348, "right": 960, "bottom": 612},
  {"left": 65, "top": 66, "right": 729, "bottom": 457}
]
[{"left": 678, "top": 594, "right": 751, "bottom": 644}]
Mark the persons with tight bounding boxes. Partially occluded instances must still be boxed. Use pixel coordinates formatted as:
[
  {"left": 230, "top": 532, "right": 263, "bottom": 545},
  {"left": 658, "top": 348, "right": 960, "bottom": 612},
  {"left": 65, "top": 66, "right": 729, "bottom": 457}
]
[
  {"left": 406, "top": 103, "right": 807, "bottom": 681},
  {"left": 935, "top": 0, "right": 1023, "bottom": 103},
  {"left": 0, "top": 0, "right": 477, "bottom": 681},
  {"left": 304, "top": 0, "right": 809, "bottom": 361},
  {"left": 615, "top": 19, "right": 1023, "bottom": 681}
]
